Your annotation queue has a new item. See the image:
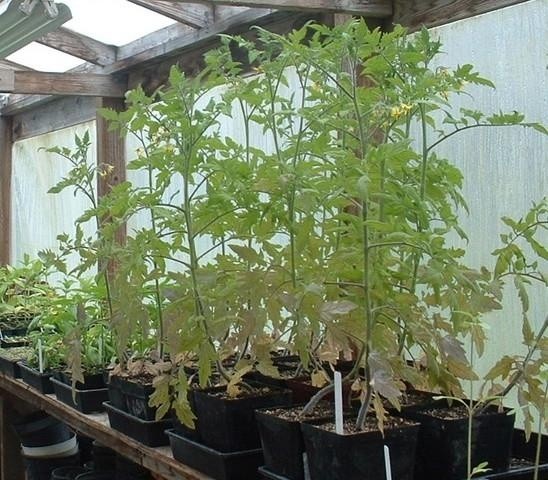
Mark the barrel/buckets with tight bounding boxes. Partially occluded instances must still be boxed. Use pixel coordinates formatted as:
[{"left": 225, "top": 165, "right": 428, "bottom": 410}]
[{"left": 10, "top": 410, "right": 153, "bottom": 480}]
